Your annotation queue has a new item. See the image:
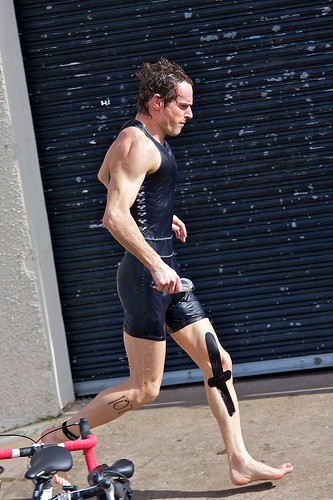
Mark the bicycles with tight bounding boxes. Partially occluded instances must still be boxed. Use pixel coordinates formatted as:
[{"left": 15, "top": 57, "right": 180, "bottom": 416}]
[{"left": 0, "top": 418, "right": 135, "bottom": 500}]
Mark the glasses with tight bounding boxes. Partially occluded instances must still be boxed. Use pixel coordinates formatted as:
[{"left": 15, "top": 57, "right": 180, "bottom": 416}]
[{"left": 150, "top": 278, "right": 195, "bottom": 296}]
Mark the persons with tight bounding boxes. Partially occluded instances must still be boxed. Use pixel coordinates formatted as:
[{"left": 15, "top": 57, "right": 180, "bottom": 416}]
[{"left": 42, "top": 60, "right": 294, "bottom": 485}]
